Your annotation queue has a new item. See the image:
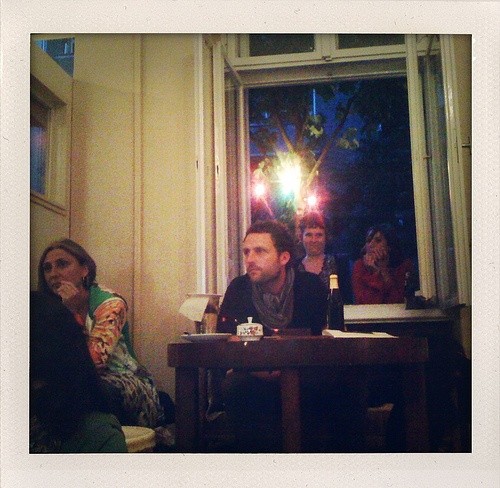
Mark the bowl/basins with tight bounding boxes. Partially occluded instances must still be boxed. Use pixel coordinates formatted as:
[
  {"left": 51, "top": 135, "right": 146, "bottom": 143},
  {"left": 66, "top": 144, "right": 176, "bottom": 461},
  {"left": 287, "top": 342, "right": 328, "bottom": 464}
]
[{"left": 236, "top": 317, "right": 264, "bottom": 341}]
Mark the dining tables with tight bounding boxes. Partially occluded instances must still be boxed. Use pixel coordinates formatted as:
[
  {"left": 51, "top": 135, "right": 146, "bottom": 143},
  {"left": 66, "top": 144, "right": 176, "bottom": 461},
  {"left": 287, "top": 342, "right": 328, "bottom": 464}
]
[{"left": 167, "top": 339, "right": 429, "bottom": 453}]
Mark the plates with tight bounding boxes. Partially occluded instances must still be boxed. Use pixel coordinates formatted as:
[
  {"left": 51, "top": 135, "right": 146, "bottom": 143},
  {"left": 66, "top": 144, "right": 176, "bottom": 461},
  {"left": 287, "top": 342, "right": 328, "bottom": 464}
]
[{"left": 182, "top": 332, "right": 232, "bottom": 346}]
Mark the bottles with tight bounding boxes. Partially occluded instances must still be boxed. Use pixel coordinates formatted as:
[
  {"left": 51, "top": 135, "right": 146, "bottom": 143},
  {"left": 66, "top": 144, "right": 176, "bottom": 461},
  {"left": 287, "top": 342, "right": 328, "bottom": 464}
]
[
  {"left": 327, "top": 274, "right": 344, "bottom": 332},
  {"left": 404, "top": 272, "right": 415, "bottom": 310}
]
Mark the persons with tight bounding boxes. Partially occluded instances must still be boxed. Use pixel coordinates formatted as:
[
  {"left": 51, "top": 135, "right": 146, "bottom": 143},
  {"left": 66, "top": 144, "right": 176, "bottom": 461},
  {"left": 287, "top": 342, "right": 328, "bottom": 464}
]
[
  {"left": 295, "top": 212, "right": 352, "bottom": 306},
  {"left": 29, "top": 291, "right": 130, "bottom": 453},
  {"left": 212, "top": 220, "right": 340, "bottom": 454},
  {"left": 38, "top": 240, "right": 165, "bottom": 427},
  {"left": 351, "top": 223, "right": 413, "bottom": 303}
]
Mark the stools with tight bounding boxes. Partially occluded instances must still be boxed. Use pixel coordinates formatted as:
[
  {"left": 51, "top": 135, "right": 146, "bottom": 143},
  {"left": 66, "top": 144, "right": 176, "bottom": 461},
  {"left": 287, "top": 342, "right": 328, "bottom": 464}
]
[{"left": 122, "top": 426, "right": 156, "bottom": 453}]
